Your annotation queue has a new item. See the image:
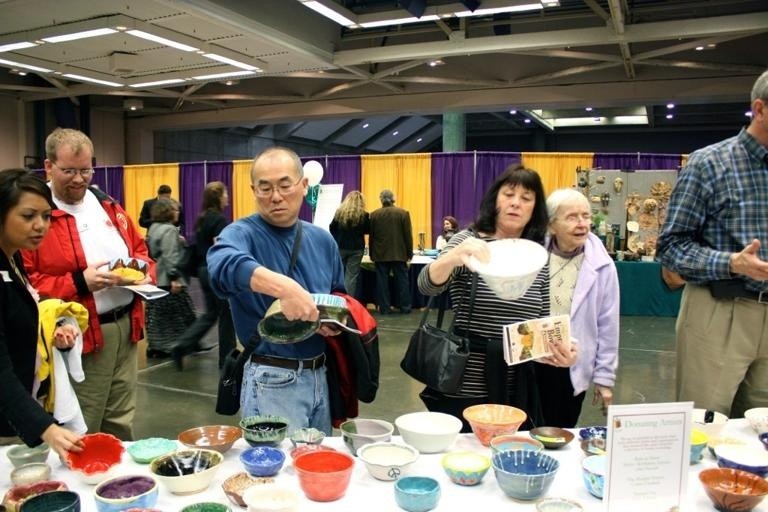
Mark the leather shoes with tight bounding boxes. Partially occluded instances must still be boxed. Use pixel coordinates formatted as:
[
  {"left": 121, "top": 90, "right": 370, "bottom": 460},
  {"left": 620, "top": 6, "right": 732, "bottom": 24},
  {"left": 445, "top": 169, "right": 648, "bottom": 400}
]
[
  {"left": 400, "top": 306, "right": 411, "bottom": 313},
  {"left": 382, "top": 307, "right": 392, "bottom": 314}
]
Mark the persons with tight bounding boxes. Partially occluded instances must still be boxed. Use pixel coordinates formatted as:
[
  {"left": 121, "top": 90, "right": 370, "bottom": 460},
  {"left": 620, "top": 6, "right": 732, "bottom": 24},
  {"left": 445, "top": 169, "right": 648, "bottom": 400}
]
[
  {"left": 417, "top": 163, "right": 577, "bottom": 433},
  {"left": 169, "top": 180, "right": 237, "bottom": 371},
  {"left": 369, "top": 190, "right": 414, "bottom": 315},
  {"left": 206, "top": 146, "right": 346, "bottom": 440},
  {"left": 436, "top": 216, "right": 461, "bottom": 251},
  {"left": 0, "top": 168, "right": 80, "bottom": 469},
  {"left": 138, "top": 184, "right": 172, "bottom": 237},
  {"left": 143, "top": 198, "right": 201, "bottom": 358},
  {"left": 655, "top": 68, "right": 768, "bottom": 415},
  {"left": 17, "top": 126, "right": 157, "bottom": 443},
  {"left": 539, "top": 186, "right": 620, "bottom": 427},
  {"left": 329, "top": 191, "right": 369, "bottom": 299}
]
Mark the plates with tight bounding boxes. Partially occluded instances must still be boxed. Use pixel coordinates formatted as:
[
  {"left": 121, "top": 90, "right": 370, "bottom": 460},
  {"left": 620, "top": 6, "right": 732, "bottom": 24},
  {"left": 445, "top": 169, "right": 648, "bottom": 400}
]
[
  {"left": 693, "top": 406, "right": 727, "bottom": 430},
  {"left": 127, "top": 437, "right": 177, "bottom": 465},
  {"left": 176, "top": 503, "right": 239, "bottom": 512},
  {"left": 291, "top": 446, "right": 335, "bottom": 461},
  {"left": 578, "top": 425, "right": 610, "bottom": 440},
  {"left": 581, "top": 441, "right": 608, "bottom": 457},
  {"left": 535, "top": 498, "right": 584, "bottom": 512}
]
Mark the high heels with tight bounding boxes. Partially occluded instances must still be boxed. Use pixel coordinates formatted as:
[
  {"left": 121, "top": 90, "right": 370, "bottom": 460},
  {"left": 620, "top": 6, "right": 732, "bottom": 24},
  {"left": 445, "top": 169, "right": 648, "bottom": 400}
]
[
  {"left": 193, "top": 344, "right": 217, "bottom": 352},
  {"left": 146, "top": 347, "right": 170, "bottom": 358}
]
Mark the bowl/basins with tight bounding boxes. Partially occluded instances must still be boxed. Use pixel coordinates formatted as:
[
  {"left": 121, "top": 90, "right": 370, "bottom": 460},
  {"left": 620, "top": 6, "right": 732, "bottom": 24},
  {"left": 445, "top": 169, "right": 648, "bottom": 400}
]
[
  {"left": 257, "top": 298, "right": 321, "bottom": 346},
  {"left": 699, "top": 468, "right": 768, "bottom": 512},
  {"left": 468, "top": 238, "right": 548, "bottom": 302},
  {"left": 294, "top": 453, "right": 355, "bottom": 501},
  {"left": 66, "top": 433, "right": 123, "bottom": 477},
  {"left": 759, "top": 433, "right": 768, "bottom": 449},
  {"left": 92, "top": 475, "right": 160, "bottom": 511},
  {"left": 712, "top": 443, "right": 768, "bottom": 477},
  {"left": 289, "top": 425, "right": 326, "bottom": 447},
  {"left": 744, "top": 407, "right": 768, "bottom": 432},
  {"left": 12, "top": 464, "right": 50, "bottom": 485},
  {"left": 223, "top": 473, "right": 276, "bottom": 505},
  {"left": 151, "top": 450, "right": 223, "bottom": 495},
  {"left": 340, "top": 418, "right": 396, "bottom": 457},
  {"left": 19, "top": 491, "right": 81, "bottom": 512},
  {"left": 396, "top": 410, "right": 463, "bottom": 453},
  {"left": 237, "top": 446, "right": 286, "bottom": 477},
  {"left": 441, "top": 453, "right": 487, "bottom": 484},
  {"left": 690, "top": 430, "right": 708, "bottom": 466},
  {"left": 489, "top": 435, "right": 544, "bottom": 455},
  {"left": 465, "top": 403, "right": 527, "bottom": 444},
  {"left": 356, "top": 442, "right": 419, "bottom": 480},
  {"left": 242, "top": 483, "right": 300, "bottom": 512},
  {"left": 394, "top": 475, "right": 439, "bottom": 512},
  {"left": 310, "top": 293, "right": 350, "bottom": 328},
  {"left": 7, "top": 442, "right": 49, "bottom": 466},
  {"left": 583, "top": 456, "right": 606, "bottom": 499},
  {"left": 529, "top": 425, "right": 575, "bottom": 449},
  {"left": 108, "top": 257, "right": 148, "bottom": 282},
  {"left": 492, "top": 450, "right": 559, "bottom": 500},
  {"left": 240, "top": 414, "right": 289, "bottom": 447},
  {"left": 178, "top": 424, "right": 240, "bottom": 454}
]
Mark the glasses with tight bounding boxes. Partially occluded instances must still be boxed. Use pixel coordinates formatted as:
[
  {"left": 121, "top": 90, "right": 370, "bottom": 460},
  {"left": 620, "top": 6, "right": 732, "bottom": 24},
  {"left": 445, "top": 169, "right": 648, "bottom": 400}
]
[
  {"left": 51, "top": 161, "right": 94, "bottom": 177},
  {"left": 248, "top": 175, "right": 304, "bottom": 198}
]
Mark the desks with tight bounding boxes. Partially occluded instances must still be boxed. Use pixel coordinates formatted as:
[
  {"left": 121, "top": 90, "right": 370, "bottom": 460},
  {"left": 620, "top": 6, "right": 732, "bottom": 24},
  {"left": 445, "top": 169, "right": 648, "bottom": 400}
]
[
  {"left": 358, "top": 250, "right": 453, "bottom": 312},
  {"left": 612, "top": 258, "right": 683, "bottom": 316},
  {"left": 0, "top": 420, "right": 768, "bottom": 512}
]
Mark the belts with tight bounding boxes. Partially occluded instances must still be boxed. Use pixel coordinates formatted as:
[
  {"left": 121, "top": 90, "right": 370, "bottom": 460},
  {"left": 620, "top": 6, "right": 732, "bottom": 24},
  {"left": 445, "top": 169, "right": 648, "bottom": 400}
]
[
  {"left": 98, "top": 296, "right": 138, "bottom": 323},
  {"left": 252, "top": 354, "right": 326, "bottom": 373},
  {"left": 709, "top": 278, "right": 768, "bottom": 304}
]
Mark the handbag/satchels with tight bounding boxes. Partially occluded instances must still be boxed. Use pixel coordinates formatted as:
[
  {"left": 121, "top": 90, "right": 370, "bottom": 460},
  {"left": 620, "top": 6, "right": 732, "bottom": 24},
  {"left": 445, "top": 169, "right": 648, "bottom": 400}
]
[
  {"left": 399, "top": 228, "right": 480, "bottom": 395},
  {"left": 213, "top": 220, "right": 302, "bottom": 416}
]
